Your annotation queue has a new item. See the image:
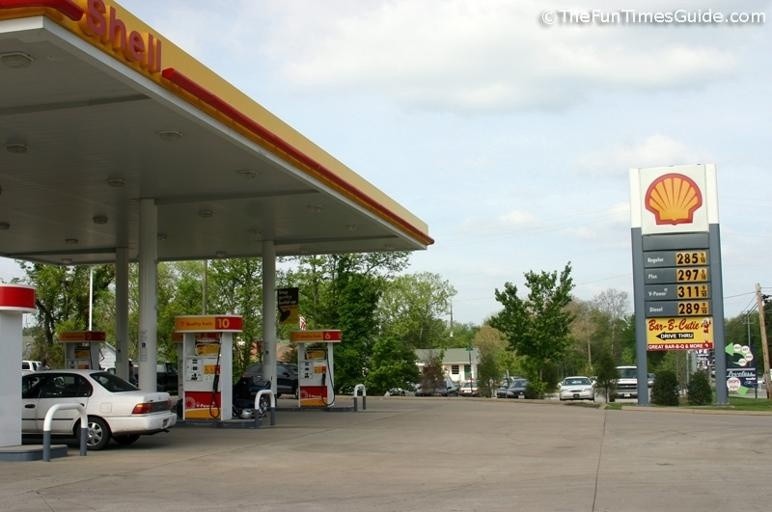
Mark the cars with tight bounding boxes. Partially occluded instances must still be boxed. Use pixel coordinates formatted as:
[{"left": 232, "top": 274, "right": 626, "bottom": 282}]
[
  {"left": 648, "top": 373, "right": 657, "bottom": 387},
  {"left": 22, "top": 360, "right": 177, "bottom": 451},
  {"left": 243, "top": 364, "right": 298, "bottom": 398},
  {"left": 558, "top": 375, "right": 597, "bottom": 400},
  {"left": 390, "top": 375, "right": 530, "bottom": 398}
]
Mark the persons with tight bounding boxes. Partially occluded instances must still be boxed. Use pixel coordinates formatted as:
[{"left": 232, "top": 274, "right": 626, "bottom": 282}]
[{"left": 37, "top": 357, "right": 49, "bottom": 370}]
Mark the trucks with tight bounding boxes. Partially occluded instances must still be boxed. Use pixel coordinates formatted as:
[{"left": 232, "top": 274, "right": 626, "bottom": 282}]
[{"left": 609, "top": 365, "right": 637, "bottom": 402}]
